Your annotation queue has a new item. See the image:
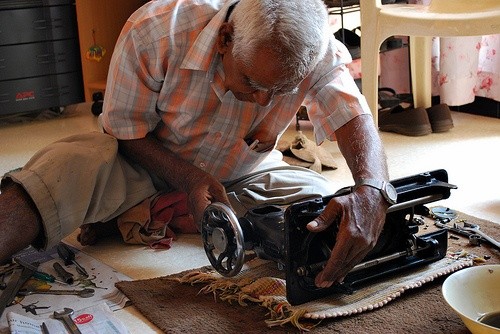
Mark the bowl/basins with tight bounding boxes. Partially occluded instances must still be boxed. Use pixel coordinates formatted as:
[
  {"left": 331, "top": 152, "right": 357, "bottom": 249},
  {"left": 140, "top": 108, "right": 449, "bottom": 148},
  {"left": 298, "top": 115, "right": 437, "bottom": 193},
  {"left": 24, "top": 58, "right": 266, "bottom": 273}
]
[{"left": 442, "top": 265, "right": 500, "bottom": 334}]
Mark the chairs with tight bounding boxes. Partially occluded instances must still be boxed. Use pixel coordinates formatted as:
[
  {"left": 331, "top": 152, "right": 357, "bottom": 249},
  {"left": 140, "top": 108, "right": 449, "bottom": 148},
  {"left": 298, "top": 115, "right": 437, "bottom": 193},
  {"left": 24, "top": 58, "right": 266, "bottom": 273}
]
[{"left": 359, "top": 0, "right": 500, "bottom": 125}]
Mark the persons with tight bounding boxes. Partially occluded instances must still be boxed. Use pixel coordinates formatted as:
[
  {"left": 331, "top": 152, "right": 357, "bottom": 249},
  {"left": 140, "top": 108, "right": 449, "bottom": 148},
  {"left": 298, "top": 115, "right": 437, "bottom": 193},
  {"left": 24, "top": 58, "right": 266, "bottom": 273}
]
[{"left": 0, "top": 0, "right": 398, "bottom": 288}]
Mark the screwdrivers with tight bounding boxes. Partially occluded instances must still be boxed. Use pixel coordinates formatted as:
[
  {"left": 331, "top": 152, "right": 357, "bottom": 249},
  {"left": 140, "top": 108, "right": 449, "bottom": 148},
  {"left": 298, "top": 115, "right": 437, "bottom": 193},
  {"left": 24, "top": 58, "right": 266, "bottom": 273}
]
[
  {"left": 8, "top": 254, "right": 38, "bottom": 272},
  {"left": 33, "top": 271, "right": 68, "bottom": 286},
  {"left": 57, "top": 245, "right": 88, "bottom": 277}
]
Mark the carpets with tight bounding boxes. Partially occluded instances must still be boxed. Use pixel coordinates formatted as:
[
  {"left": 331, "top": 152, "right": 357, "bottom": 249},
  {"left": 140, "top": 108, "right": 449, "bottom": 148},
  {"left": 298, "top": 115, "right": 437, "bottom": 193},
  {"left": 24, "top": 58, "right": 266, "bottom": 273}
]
[{"left": 113, "top": 205, "right": 500, "bottom": 334}]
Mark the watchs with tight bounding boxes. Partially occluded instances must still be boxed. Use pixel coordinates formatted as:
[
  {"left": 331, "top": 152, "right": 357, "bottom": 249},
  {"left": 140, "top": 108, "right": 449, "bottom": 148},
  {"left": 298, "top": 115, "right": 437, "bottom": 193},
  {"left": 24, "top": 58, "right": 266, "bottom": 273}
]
[{"left": 350, "top": 178, "right": 397, "bottom": 206}]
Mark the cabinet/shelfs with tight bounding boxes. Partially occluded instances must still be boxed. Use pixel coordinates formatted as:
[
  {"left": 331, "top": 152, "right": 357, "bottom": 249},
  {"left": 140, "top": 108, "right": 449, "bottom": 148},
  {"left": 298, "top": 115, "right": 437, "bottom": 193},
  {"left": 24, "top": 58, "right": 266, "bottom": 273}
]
[
  {"left": 0, "top": 0, "right": 85, "bottom": 121},
  {"left": 295, "top": 0, "right": 410, "bottom": 131}
]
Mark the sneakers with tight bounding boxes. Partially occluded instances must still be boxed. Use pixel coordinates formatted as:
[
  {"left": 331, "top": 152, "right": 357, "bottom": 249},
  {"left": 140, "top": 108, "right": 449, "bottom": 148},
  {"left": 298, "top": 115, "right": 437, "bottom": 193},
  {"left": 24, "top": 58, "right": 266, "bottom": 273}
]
[
  {"left": 378, "top": 104, "right": 432, "bottom": 136},
  {"left": 426, "top": 104, "right": 454, "bottom": 133}
]
